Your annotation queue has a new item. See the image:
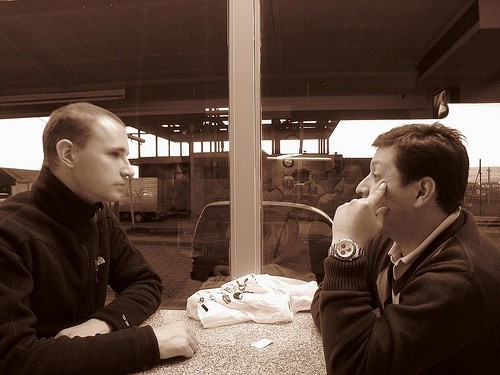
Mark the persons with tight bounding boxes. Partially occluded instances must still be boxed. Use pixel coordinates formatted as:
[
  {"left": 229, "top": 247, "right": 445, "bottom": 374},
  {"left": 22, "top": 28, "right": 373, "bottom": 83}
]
[
  {"left": 307, "top": 160, "right": 372, "bottom": 285},
  {"left": 0, "top": 101, "right": 198, "bottom": 375},
  {"left": 309, "top": 123, "right": 500, "bottom": 375}
]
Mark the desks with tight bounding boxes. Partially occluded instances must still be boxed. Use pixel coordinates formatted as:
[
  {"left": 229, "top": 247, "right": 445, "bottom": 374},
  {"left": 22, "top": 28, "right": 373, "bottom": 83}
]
[{"left": 133, "top": 309, "right": 327, "bottom": 375}]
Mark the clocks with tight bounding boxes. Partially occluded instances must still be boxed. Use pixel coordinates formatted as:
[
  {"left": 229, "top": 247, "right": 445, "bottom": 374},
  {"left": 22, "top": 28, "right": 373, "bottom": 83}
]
[{"left": 283, "top": 159, "right": 294, "bottom": 168}]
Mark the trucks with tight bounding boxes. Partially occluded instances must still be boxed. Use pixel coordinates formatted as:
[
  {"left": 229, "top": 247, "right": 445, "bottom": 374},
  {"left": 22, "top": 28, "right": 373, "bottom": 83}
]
[{"left": 115, "top": 174, "right": 173, "bottom": 222}]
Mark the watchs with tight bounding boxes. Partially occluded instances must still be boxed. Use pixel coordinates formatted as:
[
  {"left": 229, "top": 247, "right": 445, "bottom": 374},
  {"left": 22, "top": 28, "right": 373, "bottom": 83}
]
[{"left": 327, "top": 238, "right": 367, "bottom": 262}]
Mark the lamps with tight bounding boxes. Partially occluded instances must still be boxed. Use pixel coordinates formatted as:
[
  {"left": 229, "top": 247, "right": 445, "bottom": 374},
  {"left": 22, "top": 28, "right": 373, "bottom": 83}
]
[{"left": 0, "top": 89, "right": 125, "bottom": 106}]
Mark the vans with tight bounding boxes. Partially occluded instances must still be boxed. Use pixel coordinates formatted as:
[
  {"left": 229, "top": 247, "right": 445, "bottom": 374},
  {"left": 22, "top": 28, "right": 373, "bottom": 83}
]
[{"left": 190, "top": 201, "right": 333, "bottom": 284}]
